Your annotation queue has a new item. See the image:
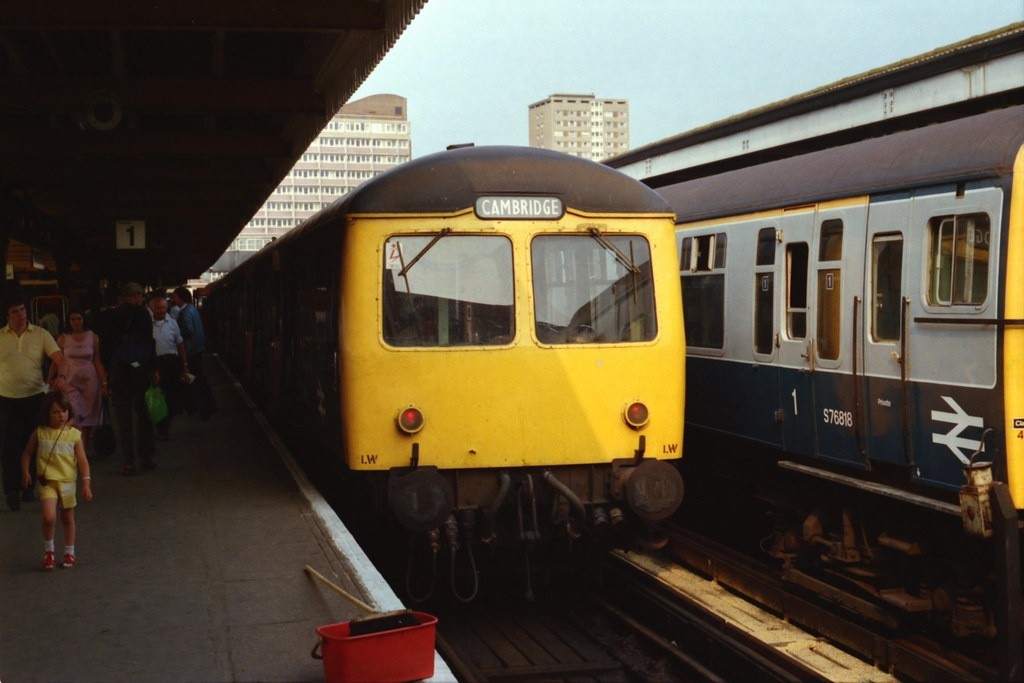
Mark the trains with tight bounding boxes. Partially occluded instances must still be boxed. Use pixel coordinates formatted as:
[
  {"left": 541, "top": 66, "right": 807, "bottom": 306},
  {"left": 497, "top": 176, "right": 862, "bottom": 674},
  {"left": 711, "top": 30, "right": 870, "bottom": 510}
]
[
  {"left": 194, "top": 143, "right": 685, "bottom": 601},
  {"left": 652, "top": 105, "right": 1024, "bottom": 650}
]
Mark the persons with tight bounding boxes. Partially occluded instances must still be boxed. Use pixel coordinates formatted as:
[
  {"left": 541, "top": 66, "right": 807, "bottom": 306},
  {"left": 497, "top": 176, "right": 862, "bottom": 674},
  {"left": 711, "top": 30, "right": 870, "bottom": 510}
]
[{"left": 0, "top": 281, "right": 218, "bottom": 570}]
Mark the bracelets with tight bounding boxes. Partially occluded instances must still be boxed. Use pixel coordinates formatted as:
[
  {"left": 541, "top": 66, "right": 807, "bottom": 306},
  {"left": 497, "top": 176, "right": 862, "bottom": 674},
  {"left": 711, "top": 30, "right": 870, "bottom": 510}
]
[
  {"left": 57, "top": 375, "right": 65, "bottom": 379},
  {"left": 82, "top": 477, "right": 91, "bottom": 480},
  {"left": 102, "top": 383, "right": 108, "bottom": 384},
  {"left": 183, "top": 361, "right": 189, "bottom": 366}
]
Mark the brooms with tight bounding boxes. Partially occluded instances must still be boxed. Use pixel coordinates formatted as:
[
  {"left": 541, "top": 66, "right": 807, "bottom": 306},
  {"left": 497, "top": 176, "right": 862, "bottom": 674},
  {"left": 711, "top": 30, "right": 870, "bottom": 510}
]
[{"left": 303, "top": 565, "right": 421, "bottom": 636}]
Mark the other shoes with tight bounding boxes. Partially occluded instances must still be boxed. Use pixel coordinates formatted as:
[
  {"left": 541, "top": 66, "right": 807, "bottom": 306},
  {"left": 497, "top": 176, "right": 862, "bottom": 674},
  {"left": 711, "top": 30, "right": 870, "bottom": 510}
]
[
  {"left": 123, "top": 464, "right": 136, "bottom": 475},
  {"left": 158, "top": 432, "right": 170, "bottom": 440},
  {"left": 140, "top": 455, "right": 152, "bottom": 469},
  {"left": 40, "top": 551, "right": 57, "bottom": 570},
  {"left": 7, "top": 494, "right": 20, "bottom": 512},
  {"left": 20, "top": 489, "right": 34, "bottom": 501},
  {"left": 61, "top": 554, "right": 75, "bottom": 567},
  {"left": 200, "top": 405, "right": 217, "bottom": 420}
]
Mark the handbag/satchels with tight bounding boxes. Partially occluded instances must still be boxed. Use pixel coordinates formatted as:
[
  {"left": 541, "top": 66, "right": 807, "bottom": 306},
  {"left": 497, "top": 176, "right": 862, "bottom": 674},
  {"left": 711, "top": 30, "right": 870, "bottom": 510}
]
[
  {"left": 93, "top": 398, "right": 116, "bottom": 456},
  {"left": 144, "top": 384, "right": 168, "bottom": 423}
]
